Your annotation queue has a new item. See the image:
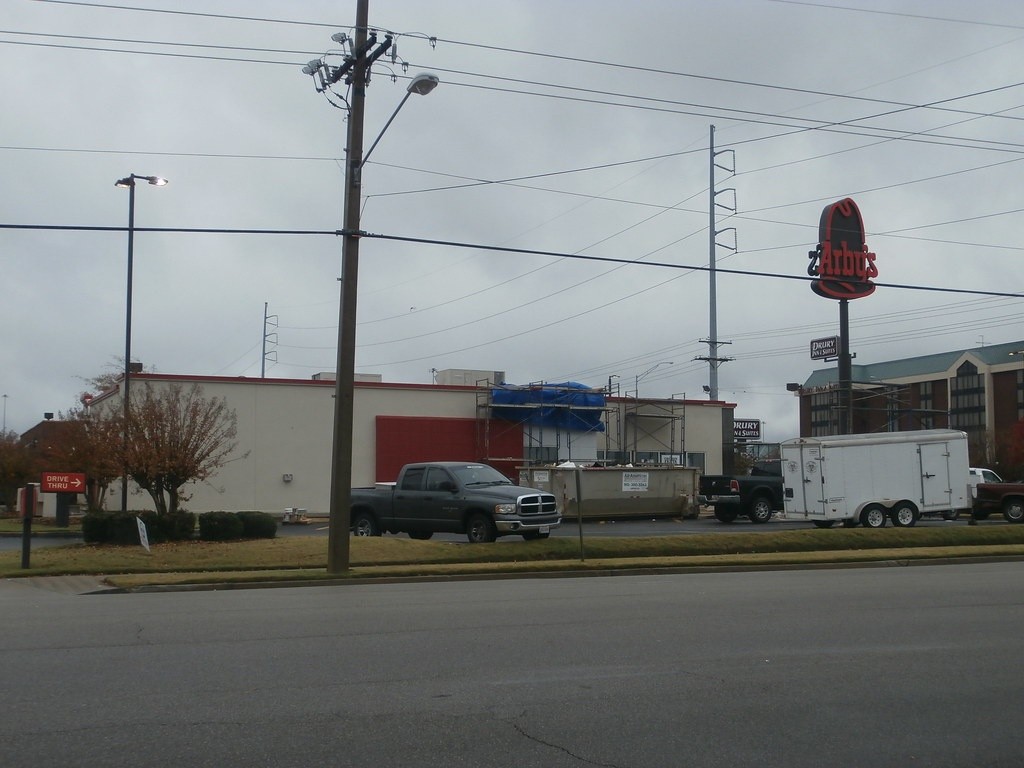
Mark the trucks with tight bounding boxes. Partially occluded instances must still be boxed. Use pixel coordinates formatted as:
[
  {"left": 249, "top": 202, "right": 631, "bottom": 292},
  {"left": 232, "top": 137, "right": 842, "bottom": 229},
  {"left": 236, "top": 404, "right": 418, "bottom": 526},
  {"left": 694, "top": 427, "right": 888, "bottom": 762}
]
[{"left": 779, "top": 428, "right": 1002, "bottom": 529}]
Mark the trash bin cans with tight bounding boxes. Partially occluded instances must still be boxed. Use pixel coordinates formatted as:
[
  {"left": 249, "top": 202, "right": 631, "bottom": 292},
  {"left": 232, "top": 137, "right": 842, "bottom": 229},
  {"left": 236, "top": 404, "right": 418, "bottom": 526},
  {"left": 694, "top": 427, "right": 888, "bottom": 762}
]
[{"left": 514, "top": 461, "right": 701, "bottom": 522}]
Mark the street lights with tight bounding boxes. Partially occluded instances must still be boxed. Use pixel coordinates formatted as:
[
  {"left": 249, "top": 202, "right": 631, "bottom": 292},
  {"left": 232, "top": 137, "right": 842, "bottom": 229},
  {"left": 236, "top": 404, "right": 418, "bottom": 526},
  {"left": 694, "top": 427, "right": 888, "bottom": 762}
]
[{"left": 114, "top": 172, "right": 170, "bottom": 510}]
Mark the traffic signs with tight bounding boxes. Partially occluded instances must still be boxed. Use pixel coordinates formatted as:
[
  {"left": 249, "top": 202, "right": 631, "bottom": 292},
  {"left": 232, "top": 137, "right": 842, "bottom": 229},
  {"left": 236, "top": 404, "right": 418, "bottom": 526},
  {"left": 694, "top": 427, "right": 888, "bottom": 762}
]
[{"left": 40, "top": 473, "right": 85, "bottom": 493}]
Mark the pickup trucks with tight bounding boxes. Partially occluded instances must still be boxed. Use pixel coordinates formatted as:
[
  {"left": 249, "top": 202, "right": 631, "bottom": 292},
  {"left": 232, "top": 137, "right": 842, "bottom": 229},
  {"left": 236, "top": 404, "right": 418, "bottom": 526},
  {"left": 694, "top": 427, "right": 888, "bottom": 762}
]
[
  {"left": 696, "top": 458, "right": 784, "bottom": 523},
  {"left": 976, "top": 480, "right": 1024, "bottom": 523},
  {"left": 349, "top": 461, "right": 562, "bottom": 544}
]
